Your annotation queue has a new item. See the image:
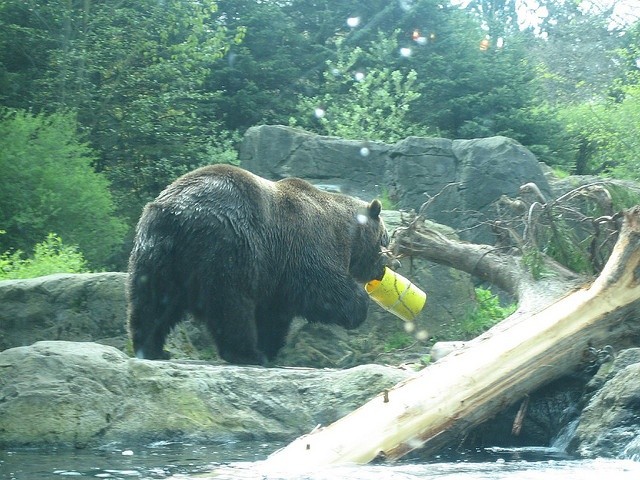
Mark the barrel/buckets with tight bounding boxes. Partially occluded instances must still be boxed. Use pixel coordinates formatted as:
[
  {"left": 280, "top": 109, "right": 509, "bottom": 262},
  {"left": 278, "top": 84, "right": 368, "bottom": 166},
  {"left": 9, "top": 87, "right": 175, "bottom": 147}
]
[{"left": 365, "top": 267, "right": 427, "bottom": 323}]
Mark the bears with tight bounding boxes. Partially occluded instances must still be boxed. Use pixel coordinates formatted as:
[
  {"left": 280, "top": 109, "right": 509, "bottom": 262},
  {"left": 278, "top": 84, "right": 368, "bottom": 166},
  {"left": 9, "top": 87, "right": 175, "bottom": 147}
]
[{"left": 126, "top": 162, "right": 403, "bottom": 365}]
